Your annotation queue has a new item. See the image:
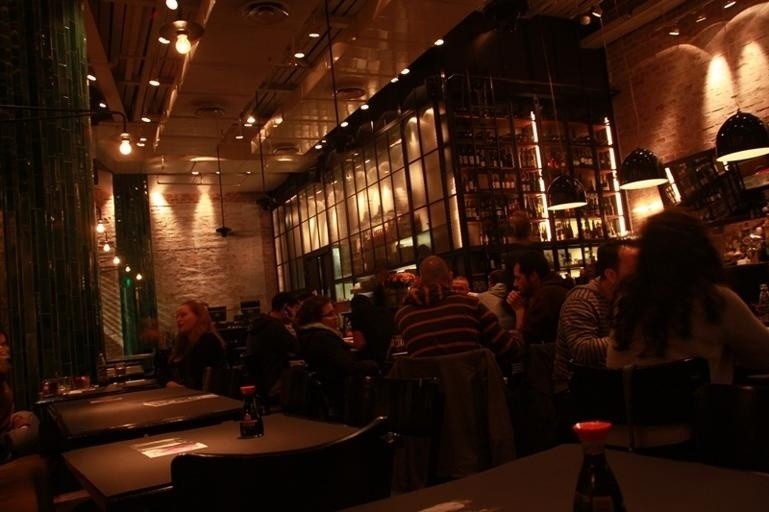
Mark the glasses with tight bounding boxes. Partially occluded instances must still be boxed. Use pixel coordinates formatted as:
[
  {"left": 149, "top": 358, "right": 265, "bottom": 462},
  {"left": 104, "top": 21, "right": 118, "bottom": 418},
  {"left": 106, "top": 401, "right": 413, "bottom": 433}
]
[{"left": 320, "top": 309, "right": 336, "bottom": 318}]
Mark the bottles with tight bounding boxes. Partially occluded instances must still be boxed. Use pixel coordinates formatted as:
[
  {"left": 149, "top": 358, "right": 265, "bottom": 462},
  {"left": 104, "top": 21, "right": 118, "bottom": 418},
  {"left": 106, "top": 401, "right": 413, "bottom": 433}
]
[
  {"left": 562, "top": 250, "right": 572, "bottom": 270},
  {"left": 540, "top": 226, "right": 549, "bottom": 241},
  {"left": 572, "top": 149, "right": 593, "bottom": 166},
  {"left": 459, "top": 148, "right": 503, "bottom": 167},
  {"left": 465, "top": 195, "right": 520, "bottom": 218},
  {"left": 606, "top": 220, "right": 618, "bottom": 237},
  {"left": 486, "top": 257, "right": 507, "bottom": 273},
  {"left": 758, "top": 283, "right": 769, "bottom": 325},
  {"left": 607, "top": 198, "right": 614, "bottom": 215},
  {"left": 493, "top": 174, "right": 515, "bottom": 189},
  {"left": 96, "top": 353, "right": 107, "bottom": 384},
  {"left": 556, "top": 222, "right": 574, "bottom": 241},
  {"left": 594, "top": 191, "right": 600, "bottom": 216},
  {"left": 463, "top": 172, "right": 477, "bottom": 192},
  {"left": 589, "top": 252, "right": 595, "bottom": 264},
  {"left": 239, "top": 385, "right": 264, "bottom": 438},
  {"left": 573, "top": 421, "right": 626, "bottom": 512},
  {"left": 599, "top": 150, "right": 611, "bottom": 169},
  {"left": 525, "top": 196, "right": 542, "bottom": 218},
  {"left": 521, "top": 172, "right": 538, "bottom": 192},
  {"left": 477, "top": 222, "right": 509, "bottom": 247},
  {"left": 580, "top": 219, "right": 605, "bottom": 239},
  {"left": 520, "top": 147, "right": 538, "bottom": 168},
  {"left": 601, "top": 175, "right": 610, "bottom": 191}
]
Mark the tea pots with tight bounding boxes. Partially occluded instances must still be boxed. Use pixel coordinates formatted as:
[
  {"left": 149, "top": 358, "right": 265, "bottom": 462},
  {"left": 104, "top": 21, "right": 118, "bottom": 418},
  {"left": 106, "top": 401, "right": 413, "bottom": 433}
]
[
  {"left": 41, "top": 380, "right": 59, "bottom": 398},
  {"left": 76, "top": 375, "right": 91, "bottom": 388}
]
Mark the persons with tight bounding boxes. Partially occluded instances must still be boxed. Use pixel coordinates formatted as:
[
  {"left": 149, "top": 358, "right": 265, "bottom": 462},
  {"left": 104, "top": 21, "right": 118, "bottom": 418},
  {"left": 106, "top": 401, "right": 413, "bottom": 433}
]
[
  {"left": 293, "top": 296, "right": 364, "bottom": 412},
  {"left": 394, "top": 256, "right": 525, "bottom": 378},
  {"left": 256, "top": 292, "right": 307, "bottom": 379},
  {"left": 161, "top": 299, "right": 225, "bottom": 390},
  {"left": 553, "top": 241, "right": 643, "bottom": 420},
  {"left": 506, "top": 253, "right": 573, "bottom": 344},
  {"left": 610, "top": 211, "right": 769, "bottom": 425},
  {"left": 451, "top": 276, "right": 478, "bottom": 298},
  {"left": 478, "top": 270, "right": 513, "bottom": 328},
  {"left": 293, "top": 288, "right": 314, "bottom": 306},
  {"left": 0, "top": 333, "right": 40, "bottom": 466}
]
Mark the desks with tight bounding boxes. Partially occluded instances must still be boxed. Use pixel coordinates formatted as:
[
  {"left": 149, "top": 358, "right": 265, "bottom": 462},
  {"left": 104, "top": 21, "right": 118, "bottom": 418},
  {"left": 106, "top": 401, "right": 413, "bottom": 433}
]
[
  {"left": 47, "top": 387, "right": 253, "bottom": 448},
  {"left": 35, "top": 378, "right": 153, "bottom": 417},
  {"left": 337, "top": 443, "right": 765, "bottom": 512},
  {"left": 105, "top": 364, "right": 144, "bottom": 382},
  {"left": 107, "top": 352, "right": 154, "bottom": 369},
  {"left": 61, "top": 416, "right": 359, "bottom": 512}
]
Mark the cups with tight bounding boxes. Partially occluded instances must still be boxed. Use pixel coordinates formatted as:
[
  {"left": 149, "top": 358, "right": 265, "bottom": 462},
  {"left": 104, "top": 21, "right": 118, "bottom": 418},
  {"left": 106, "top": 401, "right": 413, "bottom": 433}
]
[{"left": 114, "top": 362, "right": 127, "bottom": 386}]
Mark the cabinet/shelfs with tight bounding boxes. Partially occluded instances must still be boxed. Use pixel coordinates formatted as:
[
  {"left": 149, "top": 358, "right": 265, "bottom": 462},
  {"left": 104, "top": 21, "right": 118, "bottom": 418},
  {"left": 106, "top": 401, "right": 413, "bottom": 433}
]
[{"left": 449, "top": 106, "right": 626, "bottom": 279}]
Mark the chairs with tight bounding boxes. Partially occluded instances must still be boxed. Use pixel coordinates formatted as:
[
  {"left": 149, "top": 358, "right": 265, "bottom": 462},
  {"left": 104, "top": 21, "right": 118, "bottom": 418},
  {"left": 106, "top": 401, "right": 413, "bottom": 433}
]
[
  {"left": 171, "top": 415, "right": 391, "bottom": 512},
  {"left": 279, "top": 372, "right": 333, "bottom": 420},
  {"left": 525, "top": 342, "right": 556, "bottom": 450},
  {"left": 358, "top": 376, "right": 447, "bottom": 492},
  {"left": 567, "top": 354, "right": 712, "bottom": 463},
  {"left": 393, "top": 348, "right": 506, "bottom": 470}
]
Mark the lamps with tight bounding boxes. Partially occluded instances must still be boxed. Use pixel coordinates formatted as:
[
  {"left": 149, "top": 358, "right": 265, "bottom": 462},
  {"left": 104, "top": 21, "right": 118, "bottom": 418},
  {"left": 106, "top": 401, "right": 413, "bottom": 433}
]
[
  {"left": 434, "top": 38, "right": 444, "bottom": 46},
  {"left": 529, "top": 112, "right": 553, "bottom": 242},
  {"left": 361, "top": 105, "right": 369, "bottom": 110},
  {"left": 216, "top": 171, "right": 220, "bottom": 174},
  {"left": 315, "top": 145, "right": 322, "bottom": 149},
  {"left": 149, "top": 78, "right": 160, "bottom": 86},
  {"left": 103, "top": 241, "right": 111, "bottom": 253},
  {"left": 86, "top": 74, "right": 97, "bottom": 82},
  {"left": 141, "top": 113, "right": 152, "bottom": 123},
  {"left": 117, "top": 132, "right": 135, "bottom": 159},
  {"left": 391, "top": 77, "right": 399, "bottom": 83},
  {"left": 548, "top": 175, "right": 588, "bottom": 212},
  {"left": 173, "top": 29, "right": 193, "bottom": 57},
  {"left": 192, "top": 171, "right": 199, "bottom": 175},
  {"left": 112, "top": 256, "right": 121, "bottom": 266},
  {"left": 95, "top": 218, "right": 106, "bottom": 235},
  {"left": 137, "top": 143, "right": 146, "bottom": 147},
  {"left": 723, "top": 1, "right": 737, "bottom": 9},
  {"left": 604, "top": 117, "right": 625, "bottom": 232},
  {"left": 332, "top": 133, "right": 353, "bottom": 153},
  {"left": 309, "top": 31, "right": 320, "bottom": 39},
  {"left": 173, "top": 19, "right": 188, "bottom": 30},
  {"left": 158, "top": 34, "right": 172, "bottom": 45},
  {"left": 322, "top": 139, "right": 326, "bottom": 143},
  {"left": 695, "top": 12, "right": 706, "bottom": 23},
  {"left": 166, "top": 0, "right": 179, "bottom": 11},
  {"left": 669, "top": 23, "right": 680, "bottom": 35},
  {"left": 139, "top": 137, "right": 148, "bottom": 141},
  {"left": 217, "top": 226, "right": 232, "bottom": 237},
  {"left": 135, "top": 273, "right": 143, "bottom": 281},
  {"left": 99, "top": 102, "right": 107, "bottom": 109},
  {"left": 294, "top": 53, "right": 305, "bottom": 59},
  {"left": 244, "top": 124, "right": 253, "bottom": 127},
  {"left": 400, "top": 68, "right": 410, "bottom": 75},
  {"left": 125, "top": 264, "right": 131, "bottom": 272},
  {"left": 272, "top": 145, "right": 299, "bottom": 161},
  {"left": 716, "top": 113, "right": 769, "bottom": 165},
  {"left": 241, "top": 0, "right": 289, "bottom": 24},
  {"left": 193, "top": 104, "right": 226, "bottom": 119},
  {"left": 619, "top": 148, "right": 669, "bottom": 194},
  {"left": 340, "top": 121, "right": 348, "bottom": 127},
  {"left": 665, "top": 167, "right": 681, "bottom": 201},
  {"left": 247, "top": 115, "right": 256, "bottom": 124},
  {"left": 256, "top": 195, "right": 278, "bottom": 211},
  {"left": 592, "top": 7, "right": 602, "bottom": 18},
  {"left": 332, "top": 86, "right": 366, "bottom": 100},
  {"left": 235, "top": 135, "right": 244, "bottom": 139},
  {"left": 247, "top": 171, "right": 251, "bottom": 174}
]
